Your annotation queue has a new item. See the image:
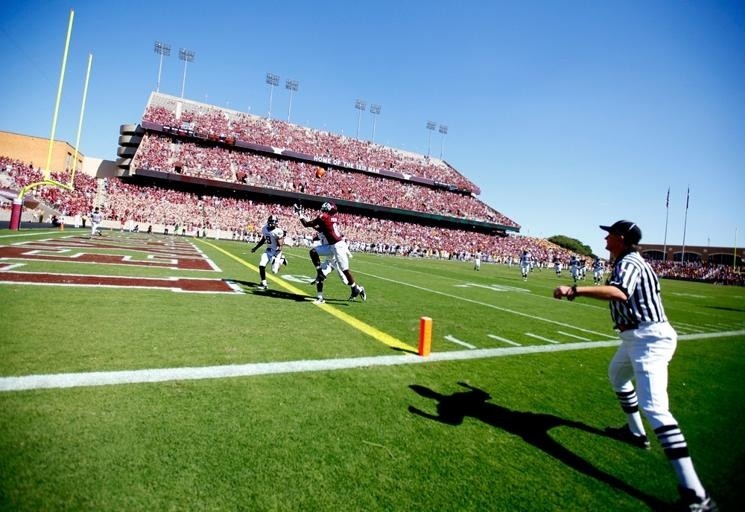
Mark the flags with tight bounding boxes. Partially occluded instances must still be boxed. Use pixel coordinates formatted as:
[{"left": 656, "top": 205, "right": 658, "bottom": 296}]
[
  {"left": 686, "top": 188, "right": 689, "bottom": 209},
  {"left": 666, "top": 187, "right": 670, "bottom": 208}
]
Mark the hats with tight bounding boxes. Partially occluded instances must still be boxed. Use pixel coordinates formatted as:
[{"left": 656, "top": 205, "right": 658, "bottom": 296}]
[{"left": 600, "top": 220, "right": 641, "bottom": 244}]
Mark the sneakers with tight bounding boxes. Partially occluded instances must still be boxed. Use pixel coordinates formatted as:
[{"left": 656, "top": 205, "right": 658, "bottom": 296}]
[
  {"left": 257, "top": 280, "right": 268, "bottom": 290},
  {"left": 311, "top": 298, "right": 326, "bottom": 304},
  {"left": 673, "top": 485, "right": 719, "bottom": 512},
  {"left": 606, "top": 424, "right": 651, "bottom": 450},
  {"left": 281, "top": 254, "right": 288, "bottom": 266},
  {"left": 311, "top": 268, "right": 326, "bottom": 285},
  {"left": 348, "top": 283, "right": 366, "bottom": 301}
]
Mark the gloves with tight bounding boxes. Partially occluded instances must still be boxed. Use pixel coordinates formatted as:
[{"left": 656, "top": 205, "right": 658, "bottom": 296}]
[
  {"left": 269, "top": 256, "right": 275, "bottom": 265},
  {"left": 293, "top": 203, "right": 302, "bottom": 219}
]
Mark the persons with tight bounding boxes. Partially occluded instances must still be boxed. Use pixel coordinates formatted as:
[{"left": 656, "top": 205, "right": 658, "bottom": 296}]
[
  {"left": 473, "top": 248, "right": 481, "bottom": 271},
  {"left": 553, "top": 220, "right": 719, "bottom": 511},
  {"left": 569, "top": 254, "right": 586, "bottom": 283},
  {"left": 292, "top": 202, "right": 367, "bottom": 302},
  {"left": 530, "top": 253, "right": 552, "bottom": 272},
  {"left": 251, "top": 215, "right": 287, "bottom": 290},
  {"left": 592, "top": 257, "right": 605, "bottom": 285},
  {"left": 2, "top": 105, "right": 595, "bottom": 265},
  {"left": 555, "top": 251, "right": 562, "bottom": 276},
  {"left": 644, "top": 258, "right": 745, "bottom": 286},
  {"left": 519, "top": 250, "right": 530, "bottom": 281}
]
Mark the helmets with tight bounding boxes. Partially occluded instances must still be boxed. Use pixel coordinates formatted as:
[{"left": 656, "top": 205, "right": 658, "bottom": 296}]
[
  {"left": 321, "top": 201, "right": 337, "bottom": 215},
  {"left": 267, "top": 215, "right": 279, "bottom": 229}
]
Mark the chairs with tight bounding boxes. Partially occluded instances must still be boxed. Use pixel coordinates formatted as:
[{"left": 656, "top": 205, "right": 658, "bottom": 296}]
[
  {"left": 0, "top": 95, "right": 610, "bottom": 270},
  {"left": 645, "top": 259, "right": 745, "bottom": 284}
]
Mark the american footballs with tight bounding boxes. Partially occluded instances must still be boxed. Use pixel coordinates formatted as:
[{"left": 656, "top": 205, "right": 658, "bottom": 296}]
[{"left": 316, "top": 168, "right": 325, "bottom": 178}]
